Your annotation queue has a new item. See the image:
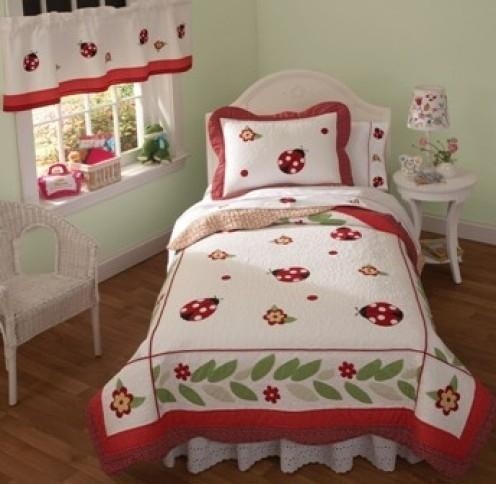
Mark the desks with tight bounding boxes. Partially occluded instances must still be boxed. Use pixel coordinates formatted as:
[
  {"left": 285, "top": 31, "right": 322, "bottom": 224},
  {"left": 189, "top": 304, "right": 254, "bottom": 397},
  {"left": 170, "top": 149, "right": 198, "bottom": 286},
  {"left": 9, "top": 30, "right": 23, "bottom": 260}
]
[{"left": 393, "top": 167, "right": 475, "bottom": 286}]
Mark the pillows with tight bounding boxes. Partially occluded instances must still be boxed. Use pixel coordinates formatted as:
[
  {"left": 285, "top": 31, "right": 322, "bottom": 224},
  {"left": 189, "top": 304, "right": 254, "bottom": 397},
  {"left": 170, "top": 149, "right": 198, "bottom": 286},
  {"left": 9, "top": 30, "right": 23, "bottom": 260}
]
[{"left": 201, "top": 98, "right": 391, "bottom": 202}]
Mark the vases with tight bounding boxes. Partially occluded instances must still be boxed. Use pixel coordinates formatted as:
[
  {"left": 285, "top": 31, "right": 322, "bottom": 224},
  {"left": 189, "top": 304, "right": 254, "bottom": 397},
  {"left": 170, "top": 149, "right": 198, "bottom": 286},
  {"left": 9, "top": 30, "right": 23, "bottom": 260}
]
[{"left": 435, "top": 162, "right": 458, "bottom": 177}]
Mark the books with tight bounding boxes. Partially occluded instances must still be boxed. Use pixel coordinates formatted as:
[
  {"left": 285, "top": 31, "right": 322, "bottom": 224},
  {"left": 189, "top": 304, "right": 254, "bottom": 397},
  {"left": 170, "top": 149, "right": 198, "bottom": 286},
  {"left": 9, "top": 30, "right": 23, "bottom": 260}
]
[{"left": 419, "top": 237, "right": 464, "bottom": 261}]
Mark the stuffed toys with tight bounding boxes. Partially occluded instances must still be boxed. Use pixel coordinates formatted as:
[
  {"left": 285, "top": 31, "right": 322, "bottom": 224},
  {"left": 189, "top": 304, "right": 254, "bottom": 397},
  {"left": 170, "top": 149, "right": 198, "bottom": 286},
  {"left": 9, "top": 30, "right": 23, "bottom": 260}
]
[
  {"left": 137, "top": 122, "right": 172, "bottom": 166},
  {"left": 68, "top": 129, "right": 115, "bottom": 165}
]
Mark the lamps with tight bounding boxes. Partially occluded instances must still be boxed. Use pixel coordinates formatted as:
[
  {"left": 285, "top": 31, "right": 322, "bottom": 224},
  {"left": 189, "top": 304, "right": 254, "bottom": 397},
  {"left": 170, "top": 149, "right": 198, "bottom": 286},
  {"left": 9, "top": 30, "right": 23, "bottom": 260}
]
[{"left": 407, "top": 79, "right": 451, "bottom": 162}]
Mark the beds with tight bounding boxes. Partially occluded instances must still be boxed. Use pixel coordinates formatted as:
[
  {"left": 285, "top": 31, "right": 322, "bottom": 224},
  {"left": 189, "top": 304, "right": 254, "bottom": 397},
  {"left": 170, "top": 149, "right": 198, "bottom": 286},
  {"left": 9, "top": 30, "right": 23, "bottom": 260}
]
[{"left": 126, "top": 61, "right": 475, "bottom": 474}]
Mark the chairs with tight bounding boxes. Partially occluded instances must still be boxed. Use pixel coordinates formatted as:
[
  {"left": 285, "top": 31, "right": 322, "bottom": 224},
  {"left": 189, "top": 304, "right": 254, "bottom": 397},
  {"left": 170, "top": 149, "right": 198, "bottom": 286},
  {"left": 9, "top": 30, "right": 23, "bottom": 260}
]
[{"left": 0, "top": 196, "right": 107, "bottom": 407}]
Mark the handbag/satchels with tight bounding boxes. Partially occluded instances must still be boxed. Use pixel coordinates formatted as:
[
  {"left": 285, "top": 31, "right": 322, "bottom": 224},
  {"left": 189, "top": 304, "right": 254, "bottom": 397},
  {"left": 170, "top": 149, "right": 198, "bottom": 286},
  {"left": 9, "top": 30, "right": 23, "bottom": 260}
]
[{"left": 38, "top": 163, "right": 83, "bottom": 199}]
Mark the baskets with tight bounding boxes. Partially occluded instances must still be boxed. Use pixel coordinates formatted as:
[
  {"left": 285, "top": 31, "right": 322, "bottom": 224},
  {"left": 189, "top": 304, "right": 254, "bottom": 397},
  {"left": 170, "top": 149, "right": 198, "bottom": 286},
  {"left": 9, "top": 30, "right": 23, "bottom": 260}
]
[{"left": 69, "top": 155, "right": 121, "bottom": 191}]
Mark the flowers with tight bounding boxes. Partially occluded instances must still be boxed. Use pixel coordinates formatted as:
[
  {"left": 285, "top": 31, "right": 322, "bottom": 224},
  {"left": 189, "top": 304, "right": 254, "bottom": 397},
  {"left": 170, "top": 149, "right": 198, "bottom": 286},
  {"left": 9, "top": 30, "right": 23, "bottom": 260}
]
[{"left": 409, "top": 136, "right": 460, "bottom": 165}]
